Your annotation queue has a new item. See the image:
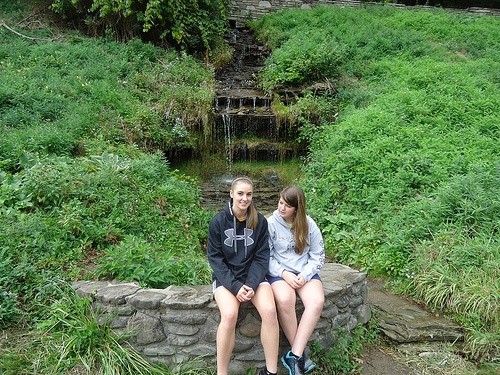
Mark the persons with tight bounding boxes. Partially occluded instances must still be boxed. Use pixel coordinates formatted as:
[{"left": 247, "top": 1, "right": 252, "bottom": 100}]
[
  {"left": 263, "top": 186, "right": 326, "bottom": 375},
  {"left": 208, "top": 177, "right": 280, "bottom": 374}
]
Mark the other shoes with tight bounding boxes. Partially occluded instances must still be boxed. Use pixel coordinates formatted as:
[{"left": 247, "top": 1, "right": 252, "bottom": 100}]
[{"left": 259, "top": 367, "right": 268, "bottom": 375}]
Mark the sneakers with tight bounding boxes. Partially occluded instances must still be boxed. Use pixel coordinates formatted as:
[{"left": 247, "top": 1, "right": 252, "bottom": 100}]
[
  {"left": 302, "top": 352, "right": 317, "bottom": 373},
  {"left": 280, "top": 350, "right": 305, "bottom": 375}
]
